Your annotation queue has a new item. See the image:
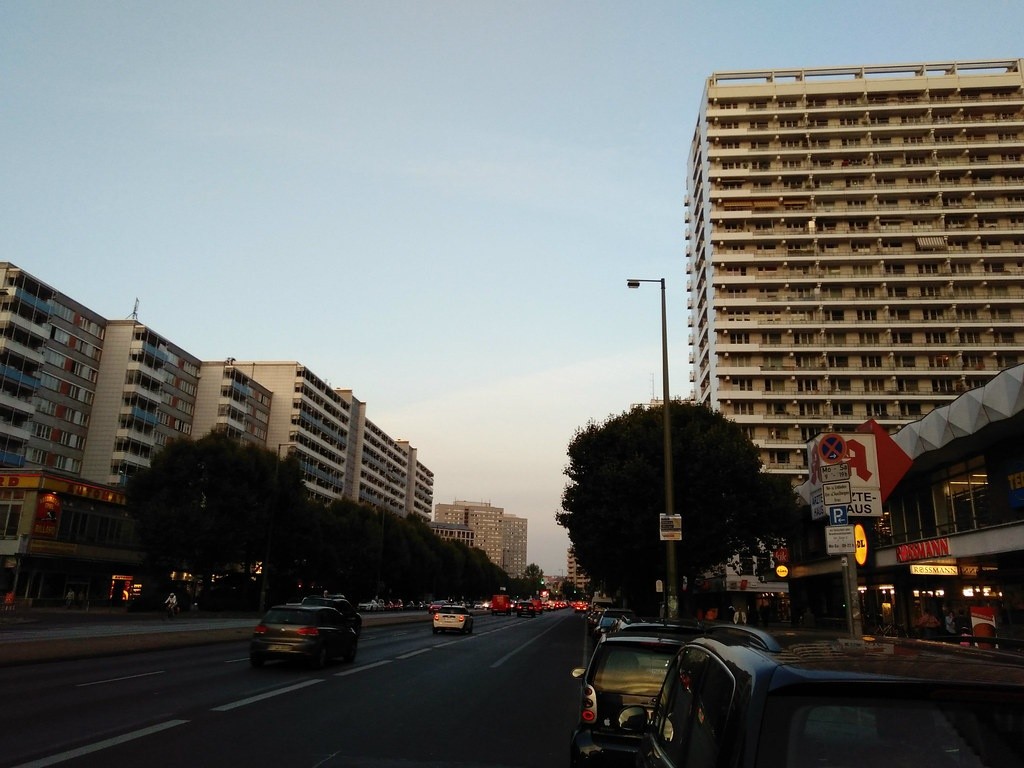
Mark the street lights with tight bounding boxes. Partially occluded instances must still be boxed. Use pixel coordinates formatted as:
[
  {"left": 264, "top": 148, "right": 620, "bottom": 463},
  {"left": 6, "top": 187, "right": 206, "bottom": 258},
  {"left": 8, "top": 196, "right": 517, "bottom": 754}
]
[{"left": 626, "top": 278, "right": 681, "bottom": 618}]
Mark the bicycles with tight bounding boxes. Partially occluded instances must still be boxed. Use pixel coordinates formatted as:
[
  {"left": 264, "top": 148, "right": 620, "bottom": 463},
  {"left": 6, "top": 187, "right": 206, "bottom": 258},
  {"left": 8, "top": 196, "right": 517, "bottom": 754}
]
[{"left": 162, "top": 602, "right": 180, "bottom": 620}]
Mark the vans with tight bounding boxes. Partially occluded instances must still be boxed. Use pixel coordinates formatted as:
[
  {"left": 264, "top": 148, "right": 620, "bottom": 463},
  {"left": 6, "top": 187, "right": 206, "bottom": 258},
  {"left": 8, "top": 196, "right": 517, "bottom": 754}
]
[
  {"left": 491, "top": 595, "right": 513, "bottom": 616},
  {"left": 592, "top": 595, "right": 614, "bottom": 608}
]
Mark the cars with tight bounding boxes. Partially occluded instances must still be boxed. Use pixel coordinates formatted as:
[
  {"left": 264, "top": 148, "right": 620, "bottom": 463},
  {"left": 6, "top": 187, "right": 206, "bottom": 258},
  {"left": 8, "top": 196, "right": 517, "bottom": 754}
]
[
  {"left": 248, "top": 589, "right": 363, "bottom": 669},
  {"left": 357, "top": 598, "right": 472, "bottom": 614},
  {"left": 432, "top": 604, "right": 474, "bottom": 635},
  {"left": 569, "top": 608, "right": 736, "bottom": 768},
  {"left": 473, "top": 598, "right": 589, "bottom": 618}
]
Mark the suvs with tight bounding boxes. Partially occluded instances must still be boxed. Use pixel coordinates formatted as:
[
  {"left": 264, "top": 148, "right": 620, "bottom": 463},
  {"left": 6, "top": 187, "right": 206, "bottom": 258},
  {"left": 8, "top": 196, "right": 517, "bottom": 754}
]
[{"left": 616, "top": 618, "right": 1024, "bottom": 768}]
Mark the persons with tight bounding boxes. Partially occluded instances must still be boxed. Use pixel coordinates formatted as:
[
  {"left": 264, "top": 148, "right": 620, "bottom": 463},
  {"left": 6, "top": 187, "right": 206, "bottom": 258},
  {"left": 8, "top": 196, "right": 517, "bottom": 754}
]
[
  {"left": 759, "top": 605, "right": 769, "bottom": 626},
  {"left": 65, "top": 589, "right": 74, "bottom": 609},
  {"left": 945, "top": 611, "right": 956, "bottom": 635},
  {"left": 919, "top": 609, "right": 941, "bottom": 626},
  {"left": 4, "top": 589, "right": 15, "bottom": 602},
  {"left": 165, "top": 592, "right": 177, "bottom": 616},
  {"left": 748, "top": 608, "right": 758, "bottom": 626},
  {"left": 322, "top": 590, "right": 330, "bottom": 597}
]
